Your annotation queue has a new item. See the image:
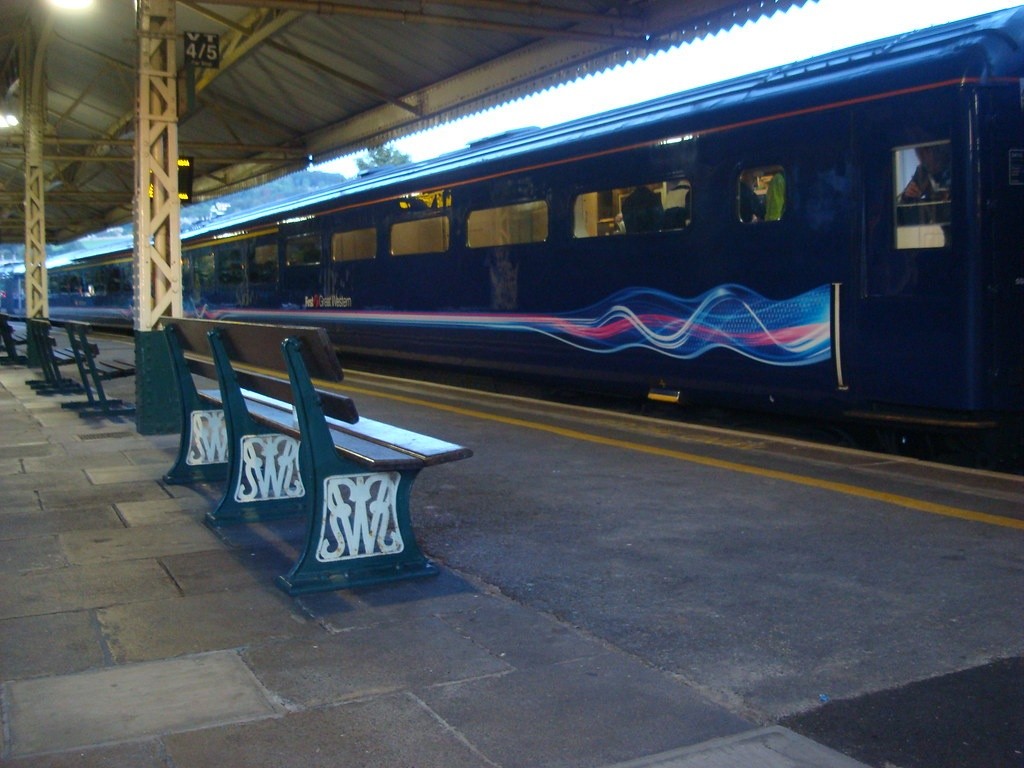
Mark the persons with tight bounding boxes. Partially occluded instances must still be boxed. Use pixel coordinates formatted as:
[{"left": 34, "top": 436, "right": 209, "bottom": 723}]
[
  {"left": 738, "top": 169, "right": 768, "bottom": 222},
  {"left": 49, "top": 229, "right": 342, "bottom": 297},
  {"left": 897, "top": 144, "right": 951, "bottom": 206},
  {"left": 620, "top": 181, "right": 665, "bottom": 233}
]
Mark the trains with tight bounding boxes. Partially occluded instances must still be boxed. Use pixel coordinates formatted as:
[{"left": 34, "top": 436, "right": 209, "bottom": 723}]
[{"left": 2, "top": 8, "right": 1021, "bottom": 456}]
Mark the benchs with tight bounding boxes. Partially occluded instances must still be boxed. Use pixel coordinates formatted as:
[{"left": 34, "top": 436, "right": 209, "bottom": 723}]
[
  {"left": 25, "top": 318, "right": 87, "bottom": 396},
  {"left": 0, "top": 313, "right": 29, "bottom": 365},
  {"left": 158, "top": 314, "right": 474, "bottom": 594},
  {"left": 60, "top": 319, "right": 136, "bottom": 419}
]
